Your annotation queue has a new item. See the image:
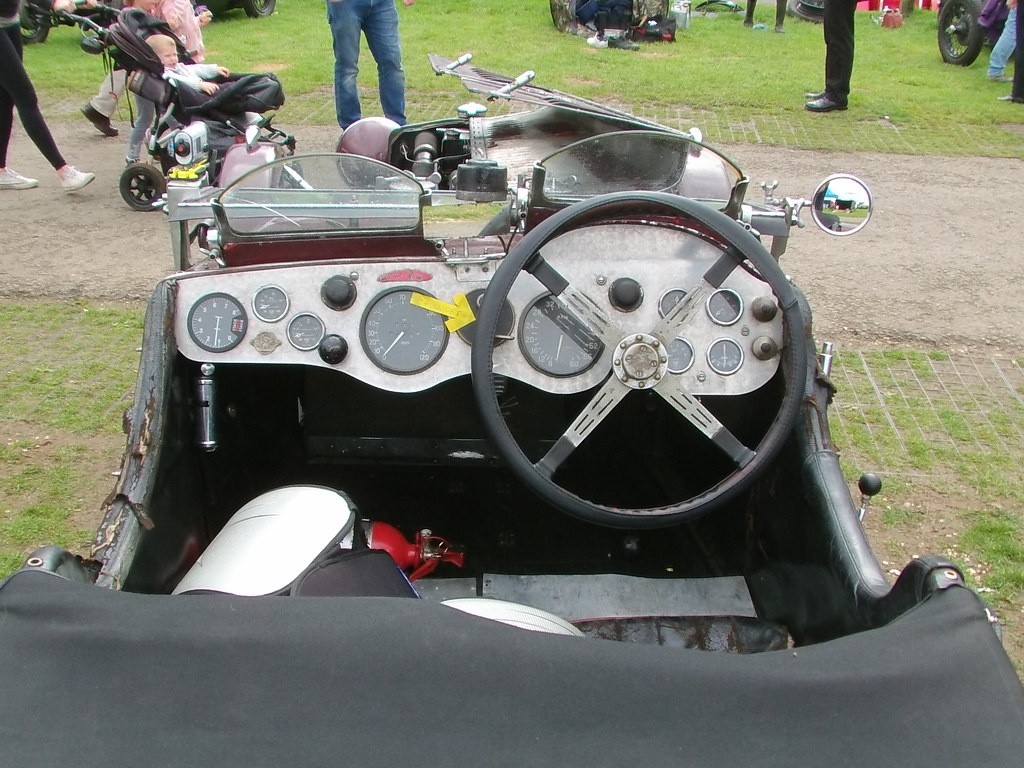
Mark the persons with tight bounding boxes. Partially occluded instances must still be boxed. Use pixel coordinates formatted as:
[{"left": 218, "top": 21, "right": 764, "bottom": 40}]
[
  {"left": 804, "top": 0, "right": 861, "bottom": 112},
  {"left": 327, "top": 0, "right": 417, "bottom": 132},
  {"left": 978, "top": 0, "right": 1024, "bottom": 104},
  {"left": 743, "top": 0, "right": 787, "bottom": 32},
  {"left": 1, "top": 0, "right": 99, "bottom": 192},
  {"left": 80, "top": 0, "right": 213, "bottom": 169},
  {"left": 145, "top": 35, "right": 263, "bottom": 126}
]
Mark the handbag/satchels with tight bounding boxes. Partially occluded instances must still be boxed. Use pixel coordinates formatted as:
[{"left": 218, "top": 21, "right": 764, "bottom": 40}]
[{"left": 629, "top": 14, "right": 678, "bottom": 43}]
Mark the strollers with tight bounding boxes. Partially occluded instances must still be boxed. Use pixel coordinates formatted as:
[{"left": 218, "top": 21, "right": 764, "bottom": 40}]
[{"left": 28, "top": 0, "right": 305, "bottom": 211}]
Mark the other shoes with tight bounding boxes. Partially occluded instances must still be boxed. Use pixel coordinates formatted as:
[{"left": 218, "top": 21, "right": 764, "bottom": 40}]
[
  {"left": 987, "top": 73, "right": 1014, "bottom": 82},
  {"left": 744, "top": 22, "right": 754, "bottom": 29},
  {"left": 776, "top": 25, "right": 785, "bottom": 33},
  {"left": 124, "top": 157, "right": 146, "bottom": 167},
  {"left": 997, "top": 95, "right": 1024, "bottom": 103},
  {"left": 587, "top": 32, "right": 607, "bottom": 49},
  {"left": 79, "top": 103, "right": 118, "bottom": 137},
  {"left": 608, "top": 36, "right": 640, "bottom": 51}
]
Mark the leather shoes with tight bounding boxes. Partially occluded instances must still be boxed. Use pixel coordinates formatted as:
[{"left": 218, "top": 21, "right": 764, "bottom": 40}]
[
  {"left": 804, "top": 97, "right": 848, "bottom": 112},
  {"left": 805, "top": 89, "right": 826, "bottom": 100}
]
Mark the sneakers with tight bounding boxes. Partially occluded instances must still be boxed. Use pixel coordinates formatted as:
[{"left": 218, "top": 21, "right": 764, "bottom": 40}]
[
  {"left": 0, "top": 167, "right": 39, "bottom": 190},
  {"left": 56, "top": 165, "right": 95, "bottom": 194}
]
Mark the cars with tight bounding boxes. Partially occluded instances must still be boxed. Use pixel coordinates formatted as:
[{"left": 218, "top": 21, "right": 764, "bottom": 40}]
[{"left": 1, "top": 106, "right": 1023, "bottom": 768}]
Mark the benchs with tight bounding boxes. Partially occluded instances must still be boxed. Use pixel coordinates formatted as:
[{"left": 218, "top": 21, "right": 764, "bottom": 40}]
[{"left": 0, "top": 540, "right": 1024, "bottom": 768}]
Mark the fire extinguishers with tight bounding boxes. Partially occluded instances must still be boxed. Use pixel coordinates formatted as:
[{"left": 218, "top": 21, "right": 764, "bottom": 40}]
[{"left": 179, "top": 518, "right": 482, "bottom": 584}]
